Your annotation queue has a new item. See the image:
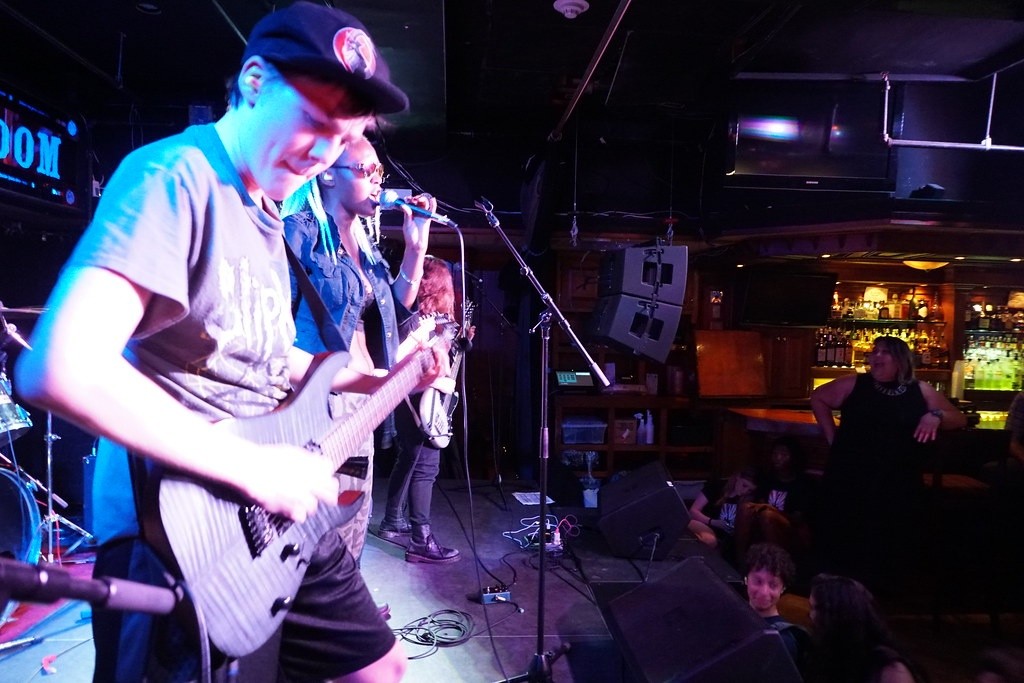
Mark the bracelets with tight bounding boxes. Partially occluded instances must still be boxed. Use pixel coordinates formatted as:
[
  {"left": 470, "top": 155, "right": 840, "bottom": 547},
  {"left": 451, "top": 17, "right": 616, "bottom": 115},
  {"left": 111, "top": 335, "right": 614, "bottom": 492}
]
[
  {"left": 398, "top": 263, "right": 424, "bottom": 285},
  {"left": 708, "top": 518, "right": 712, "bottom": 526}
]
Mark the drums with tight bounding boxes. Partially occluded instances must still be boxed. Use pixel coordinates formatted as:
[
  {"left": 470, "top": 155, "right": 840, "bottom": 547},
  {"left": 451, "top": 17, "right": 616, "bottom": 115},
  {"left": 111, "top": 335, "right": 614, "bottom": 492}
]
[
  {"left": 0, "top": 370, "right": 33, "bottom": 446},
  {"left": 0, "top": 466, "right": 43, "bottom": 627}
]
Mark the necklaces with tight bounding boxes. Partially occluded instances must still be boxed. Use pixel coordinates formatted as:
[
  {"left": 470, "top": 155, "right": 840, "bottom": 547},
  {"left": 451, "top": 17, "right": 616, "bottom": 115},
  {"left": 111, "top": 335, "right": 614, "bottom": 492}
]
[{"left": 869, "top": 379, "right": 907, "bottom": 396}]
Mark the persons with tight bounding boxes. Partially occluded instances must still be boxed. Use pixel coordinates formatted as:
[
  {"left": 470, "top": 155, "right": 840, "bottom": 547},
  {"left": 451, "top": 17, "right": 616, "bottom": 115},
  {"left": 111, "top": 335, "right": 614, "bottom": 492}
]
[
  {"left": 1003, "top": 389, "right": 1024, "bottom": 464},
  {"left": 685, "top": 436, "right": 1023, "bottom": 683},
  {"left": 811, "top": 338, "right": 967, "bottom": 607},
  {"left": 372, "top": 255, "right": 462, "bottom": 560},
  {"left": 13, "top": 7, "right": 409, "bottom": 683},
  {"left": 281, "top": 134, "right": 437, "bottom": 563}
]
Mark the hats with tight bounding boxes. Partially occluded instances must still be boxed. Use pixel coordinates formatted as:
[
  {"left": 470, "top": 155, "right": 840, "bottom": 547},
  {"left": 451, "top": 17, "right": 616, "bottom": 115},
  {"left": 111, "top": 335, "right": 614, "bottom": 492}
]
[{"left": 241, "top": 2, "right": 407, "bottom": 115}]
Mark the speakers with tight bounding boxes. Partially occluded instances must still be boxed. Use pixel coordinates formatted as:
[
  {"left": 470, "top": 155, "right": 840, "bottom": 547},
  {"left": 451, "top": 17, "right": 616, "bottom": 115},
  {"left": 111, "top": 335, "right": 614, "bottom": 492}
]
[
  {"left": 585, "top": 245, "right": 688, "bottom": 365},
  {"left": 610, "top": 554, "right": 804, "bottom": 683},
  {"left": 598, "top": 459, "right": 693, "bottom": 561}
]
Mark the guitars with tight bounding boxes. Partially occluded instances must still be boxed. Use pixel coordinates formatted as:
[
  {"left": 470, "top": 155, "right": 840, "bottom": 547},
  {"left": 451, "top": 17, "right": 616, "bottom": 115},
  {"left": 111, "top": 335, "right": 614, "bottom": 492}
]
[
  {"left": 413, "top": 297, "right": 477, "bottom": 450},
  {"left": 141, "top": 315, "right": 458, "bottom": 661}
]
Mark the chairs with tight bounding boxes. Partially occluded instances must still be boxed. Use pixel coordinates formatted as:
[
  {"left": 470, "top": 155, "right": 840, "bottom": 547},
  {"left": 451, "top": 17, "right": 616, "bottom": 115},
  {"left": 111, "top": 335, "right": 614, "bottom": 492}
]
[{"left": 902, "top": 429, "right": 1013, "bottom": 641}]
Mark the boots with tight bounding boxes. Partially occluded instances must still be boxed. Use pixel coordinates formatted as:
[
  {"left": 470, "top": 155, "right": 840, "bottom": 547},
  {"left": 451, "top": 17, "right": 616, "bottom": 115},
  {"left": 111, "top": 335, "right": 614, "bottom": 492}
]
[
  {"left": 405, "top": 524, "right": 461, "bottom": 564},
  {"left": 378, "top": 508, "right": 413, "bottom": 538}
]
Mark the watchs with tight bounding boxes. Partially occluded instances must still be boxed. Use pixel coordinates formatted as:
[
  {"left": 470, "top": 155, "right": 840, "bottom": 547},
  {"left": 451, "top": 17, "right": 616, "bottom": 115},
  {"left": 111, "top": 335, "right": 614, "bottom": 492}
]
[{"left": 929, "top": 409, "right": 943, "bottom": 423}]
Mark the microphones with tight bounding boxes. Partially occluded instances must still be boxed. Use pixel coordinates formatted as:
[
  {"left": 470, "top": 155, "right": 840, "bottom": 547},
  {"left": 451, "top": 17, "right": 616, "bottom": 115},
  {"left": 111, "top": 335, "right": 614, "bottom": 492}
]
[{"left": 380, "top": 190, "right": 458, "bottom": 229}]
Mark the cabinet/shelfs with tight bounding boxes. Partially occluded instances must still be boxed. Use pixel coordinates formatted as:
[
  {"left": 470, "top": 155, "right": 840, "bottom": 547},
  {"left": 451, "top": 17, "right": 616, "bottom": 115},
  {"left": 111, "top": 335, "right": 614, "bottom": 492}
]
[
  {"left": 550, "top": 344, "right": 718, "bottom": 477},
  {"left": 807, "top": 281, "right": 1024, "bottom": 412}
]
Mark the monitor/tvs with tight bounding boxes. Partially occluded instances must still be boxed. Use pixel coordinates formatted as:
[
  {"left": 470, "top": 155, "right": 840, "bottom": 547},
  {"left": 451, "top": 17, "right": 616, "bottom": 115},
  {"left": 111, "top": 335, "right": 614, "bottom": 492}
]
[
  {"left": 740, "top": 270, "right": 837, "bottom": 328},
  {"left": 723, "top": 78, "right": 904, "bottom": 194}
]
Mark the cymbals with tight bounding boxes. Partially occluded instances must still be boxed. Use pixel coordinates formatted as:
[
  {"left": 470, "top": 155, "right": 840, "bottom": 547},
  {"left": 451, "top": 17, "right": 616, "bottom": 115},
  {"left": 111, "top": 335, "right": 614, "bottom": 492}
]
[{"left": 0, "top": 306, "right": 44, "bottom": 313}]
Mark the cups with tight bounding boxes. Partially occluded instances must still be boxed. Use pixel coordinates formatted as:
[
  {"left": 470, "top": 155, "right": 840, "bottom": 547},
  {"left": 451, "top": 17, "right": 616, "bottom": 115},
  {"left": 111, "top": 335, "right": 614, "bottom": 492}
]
[{"left": 583, "top": 490, "right": 597, "bottom": 507}]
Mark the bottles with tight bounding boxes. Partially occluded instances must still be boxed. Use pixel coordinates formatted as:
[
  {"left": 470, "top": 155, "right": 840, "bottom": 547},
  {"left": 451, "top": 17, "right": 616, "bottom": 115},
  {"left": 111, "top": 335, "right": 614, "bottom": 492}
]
[
  {"left": 831, "top": 290, "right": 944, "bottom": 322},
  {"left": 926, "top": 379, "right": 946, "bottom": 393},
  {"left": 962, "top": 298, "right": 1024, "bottom": 390},
  {"left": 646, "top": 415, "right": 654, "bottom": 444},
  {"left": 814, "top": 327, "right": 949, "bottom": 370},
  {"left": 637, "top": 419, "right": 647, "bottom": 445}
]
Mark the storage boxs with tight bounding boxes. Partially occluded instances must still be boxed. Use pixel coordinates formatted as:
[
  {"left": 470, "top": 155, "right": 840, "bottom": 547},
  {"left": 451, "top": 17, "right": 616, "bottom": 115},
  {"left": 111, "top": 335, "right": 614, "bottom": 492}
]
[
  {"left": 561, "top": 417, "right": 607, "bottom": 444},
  {"left": 614, "top": 420, "right": 637, "bottom": 445}
]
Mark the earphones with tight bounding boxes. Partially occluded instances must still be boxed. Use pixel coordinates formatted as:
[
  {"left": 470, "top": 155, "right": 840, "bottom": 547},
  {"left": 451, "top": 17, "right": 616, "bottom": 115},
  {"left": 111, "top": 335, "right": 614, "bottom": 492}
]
[
  {"left": 246, "top": 76, "right": 260, "bottom": 92},
  {"left": 324, "top": 172, "right": 333, "bottom": 180}
]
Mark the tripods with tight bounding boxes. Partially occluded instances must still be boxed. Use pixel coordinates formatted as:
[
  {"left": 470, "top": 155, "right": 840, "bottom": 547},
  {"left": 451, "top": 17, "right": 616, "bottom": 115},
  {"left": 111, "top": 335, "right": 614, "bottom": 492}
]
[{"left": 36, "top": 410, "right": 95, "bottom": 565}]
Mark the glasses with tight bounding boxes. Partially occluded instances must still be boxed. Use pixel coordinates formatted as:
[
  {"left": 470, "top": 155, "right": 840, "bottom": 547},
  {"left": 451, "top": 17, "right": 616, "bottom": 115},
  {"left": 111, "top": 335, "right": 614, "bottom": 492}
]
[{"left": 330, "top": 163, "right": 384, "bottom": 178}]
